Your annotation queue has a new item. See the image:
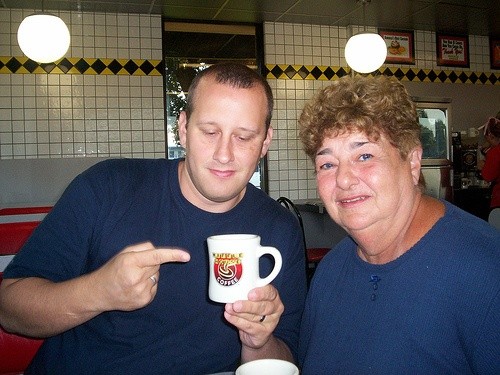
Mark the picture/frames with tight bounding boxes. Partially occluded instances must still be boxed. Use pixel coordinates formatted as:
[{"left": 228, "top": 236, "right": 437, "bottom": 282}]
[
  {"left": 489, "top": 31, "right": 500, "bottom": 70},
  {"left": 436, "top": 32, "right": 470, "bottom": 68},
  {"left": 378, "top": 28, "right": 415, "bottom": 65}
]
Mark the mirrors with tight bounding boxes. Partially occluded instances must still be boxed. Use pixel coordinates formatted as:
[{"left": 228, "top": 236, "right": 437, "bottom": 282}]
[{"left": 162, "top": 17, "right": 269, "bottom": 194}]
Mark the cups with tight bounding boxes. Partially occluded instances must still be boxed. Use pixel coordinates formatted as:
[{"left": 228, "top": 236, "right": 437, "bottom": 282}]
[
  {"left": 207, "top": 234, "right": 283, "bottom": 303},
  {"left": 235, "top": 358, "right": 299, "bottom": 375},
  {"left": 468, "top": 128, "right": 479, "bottom": 137},
  {"left": 461, "top": 177, "right": 490, "bottom": 189}
]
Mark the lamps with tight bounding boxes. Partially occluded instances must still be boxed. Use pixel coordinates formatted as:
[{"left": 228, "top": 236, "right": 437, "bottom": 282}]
[
  {"left": 16, "top": 1, "right": 71, "bottom": 65},
  {"left": 344, "top": 0, "right": 387, "bottom": 74}
]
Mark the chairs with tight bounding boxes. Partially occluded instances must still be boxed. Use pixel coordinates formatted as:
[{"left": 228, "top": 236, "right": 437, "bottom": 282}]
[{"left": 278, "top": 197, "right": 332, "bottom": 290}]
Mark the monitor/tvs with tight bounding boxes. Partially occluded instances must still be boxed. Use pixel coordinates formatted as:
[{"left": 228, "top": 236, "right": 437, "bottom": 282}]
[{"left": 415, "top": 103, "right": 452, "bottom": 166}]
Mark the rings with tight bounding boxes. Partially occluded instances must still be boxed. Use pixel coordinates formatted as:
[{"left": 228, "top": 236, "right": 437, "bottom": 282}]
[
  {"left": 258, "top": 315, "right": 266, "bottom": 323},
  {"left": 150, "top": 276, "right": 157, "bottom": 285}
]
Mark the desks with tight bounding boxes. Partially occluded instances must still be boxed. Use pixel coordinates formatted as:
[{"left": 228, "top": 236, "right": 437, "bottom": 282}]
[{"left": 293, "top": 199, "right": 327, "bottom": 214}]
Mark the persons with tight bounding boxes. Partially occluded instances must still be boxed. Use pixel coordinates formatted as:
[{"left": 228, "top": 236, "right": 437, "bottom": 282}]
[
  {"left": 0, "top": 61, "right": 306, "bottom": 375},
  {"left": 299, "top": 75, "right": 500, "bottom": 375},
  {"left": 481, "top": 119, "right": 500, "bottom": 228}
]
